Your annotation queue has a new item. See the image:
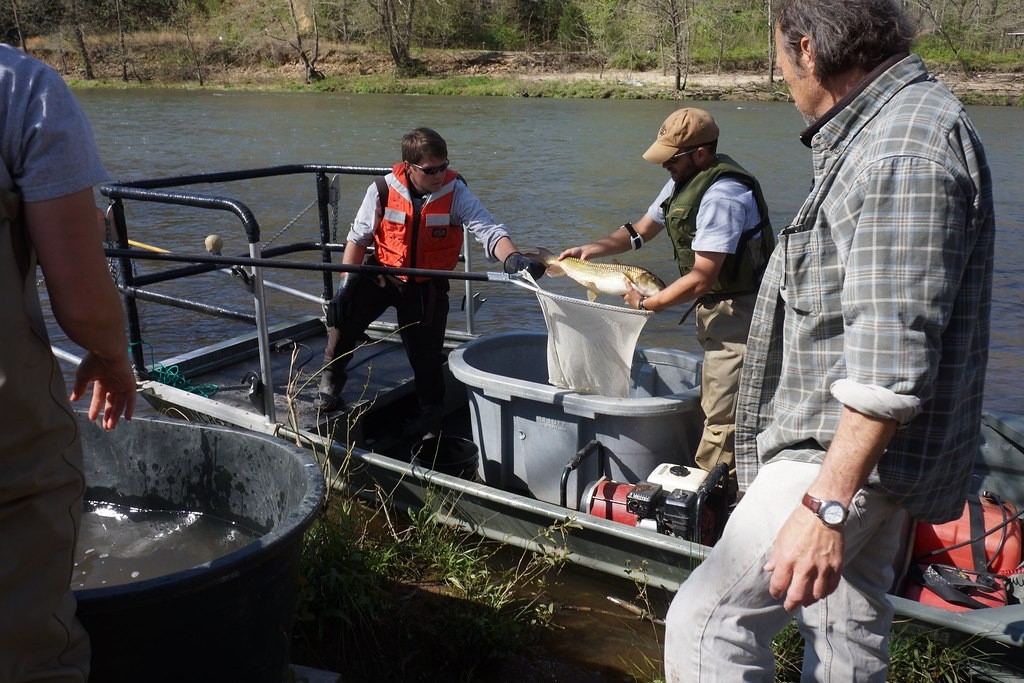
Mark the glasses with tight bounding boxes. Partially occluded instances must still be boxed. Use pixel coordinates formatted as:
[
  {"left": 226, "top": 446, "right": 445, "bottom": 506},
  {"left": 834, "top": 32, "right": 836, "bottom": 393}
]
[
  {"left": 666, "top": 145, "right": 712, "bottom": 163},
  {"left": 406, "top": 159, "right": 449, "bottom": 175}
]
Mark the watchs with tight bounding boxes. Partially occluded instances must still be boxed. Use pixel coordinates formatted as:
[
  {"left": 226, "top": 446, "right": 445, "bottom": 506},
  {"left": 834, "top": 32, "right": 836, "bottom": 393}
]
[{"left": 802, "top": 492, "right": 850, "bottom": 525}]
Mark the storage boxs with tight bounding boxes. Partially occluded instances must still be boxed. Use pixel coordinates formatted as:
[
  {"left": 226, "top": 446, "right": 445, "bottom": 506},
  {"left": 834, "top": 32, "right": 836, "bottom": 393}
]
[{"left": 447, "top": 328, "right": 704, "bottom": 510}]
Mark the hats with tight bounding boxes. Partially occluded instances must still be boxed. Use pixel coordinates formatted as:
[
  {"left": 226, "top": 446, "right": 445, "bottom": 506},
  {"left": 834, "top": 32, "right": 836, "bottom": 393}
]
[{"left": 641, "top": 107, "right": 719, "bottom": 164}]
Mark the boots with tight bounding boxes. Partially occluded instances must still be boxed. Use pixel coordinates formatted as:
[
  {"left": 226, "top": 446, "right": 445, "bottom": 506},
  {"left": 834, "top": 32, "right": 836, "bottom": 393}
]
[
  {"left": 313, "top": 324, "right": 359, "bottom": 413},
  {"left": 408, "top": 383, "right": 445, "bottom": 458}
]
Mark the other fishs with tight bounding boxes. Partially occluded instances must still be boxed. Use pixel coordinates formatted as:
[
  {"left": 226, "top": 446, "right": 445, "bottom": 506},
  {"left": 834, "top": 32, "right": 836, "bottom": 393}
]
[{"left": 521, "top": 245, "right": 667, "bottom": 303}]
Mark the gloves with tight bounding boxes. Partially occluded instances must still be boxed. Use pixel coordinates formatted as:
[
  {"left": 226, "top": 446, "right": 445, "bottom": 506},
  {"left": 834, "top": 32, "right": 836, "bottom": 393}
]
[{"left": 504, "top": 251, "right": 545, "bottom": 284}]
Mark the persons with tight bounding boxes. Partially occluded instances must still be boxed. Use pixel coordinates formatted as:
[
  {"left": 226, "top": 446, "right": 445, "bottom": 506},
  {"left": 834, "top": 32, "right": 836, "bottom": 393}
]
[
  {"left": 315, "top": 128, "right": 546, "bottom": 433},
  {"left": 0, "top": 43, "right": 139, "bottom": 683},
  {"left": 663, "top": 1, "right": 997, "bottom": 683},
  {"left": 544, "top": 107, "right": 776, "bottom": 484}
]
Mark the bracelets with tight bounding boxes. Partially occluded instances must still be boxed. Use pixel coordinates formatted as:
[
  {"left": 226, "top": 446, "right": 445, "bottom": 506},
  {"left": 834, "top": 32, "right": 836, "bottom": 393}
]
[{"left": 638, "top": 296, "right": 649, "bottom": 312}]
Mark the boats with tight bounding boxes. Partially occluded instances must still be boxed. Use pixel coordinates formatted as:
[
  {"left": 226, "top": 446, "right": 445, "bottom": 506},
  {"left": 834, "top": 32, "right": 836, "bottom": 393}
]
[{"left": 97, "top": 163, "right": 1024, "bottom": 683}]
